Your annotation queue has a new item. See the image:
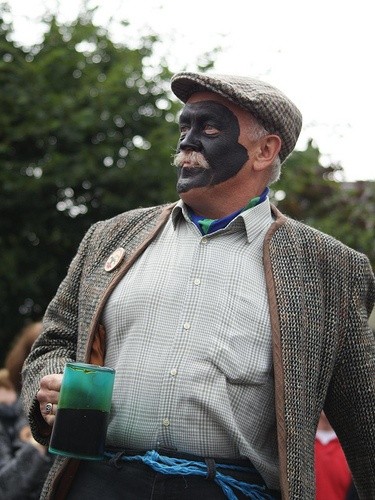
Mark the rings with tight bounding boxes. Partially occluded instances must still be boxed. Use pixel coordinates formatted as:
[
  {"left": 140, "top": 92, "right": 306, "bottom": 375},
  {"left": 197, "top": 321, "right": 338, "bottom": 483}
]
[{"left": 44, "top": 401, "right": 52, "bottom": 413}]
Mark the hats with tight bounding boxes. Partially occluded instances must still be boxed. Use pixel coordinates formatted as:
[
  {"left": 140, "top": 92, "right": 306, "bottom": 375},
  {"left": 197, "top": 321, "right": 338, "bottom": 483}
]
[{"left": 170, "top": 71, "right": 302, "bottom": 164}]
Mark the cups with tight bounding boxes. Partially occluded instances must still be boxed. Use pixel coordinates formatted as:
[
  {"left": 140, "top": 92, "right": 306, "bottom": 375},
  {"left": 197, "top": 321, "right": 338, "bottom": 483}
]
[{"left": 48, "top": 363, "right": 116, "bottom": 460}]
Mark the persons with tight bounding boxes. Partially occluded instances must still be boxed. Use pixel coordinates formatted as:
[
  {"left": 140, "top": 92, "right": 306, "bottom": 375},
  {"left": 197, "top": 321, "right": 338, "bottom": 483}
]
[
  {"left": 0, "top": 320, "right": 105, "bottom": 500},
  {"left": 20, "top": 71, "right": 375, "bottom": 499},
  {"left": 313, "top": 410, "right": 354, "bottom": 499}
]
